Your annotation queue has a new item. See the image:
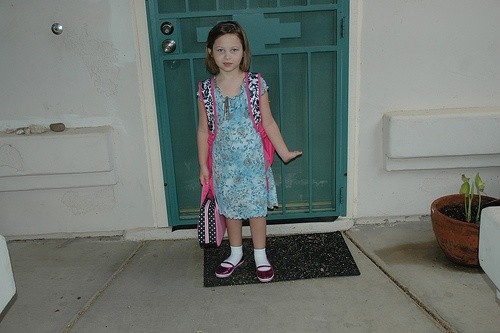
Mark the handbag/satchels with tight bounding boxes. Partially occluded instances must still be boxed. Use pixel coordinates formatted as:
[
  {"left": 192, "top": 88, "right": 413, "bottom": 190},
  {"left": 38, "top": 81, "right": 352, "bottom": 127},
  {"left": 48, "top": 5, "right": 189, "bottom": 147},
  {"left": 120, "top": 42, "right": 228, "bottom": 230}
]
[{"left": 198, "top": 180, "right": 226, "bottom": 248}]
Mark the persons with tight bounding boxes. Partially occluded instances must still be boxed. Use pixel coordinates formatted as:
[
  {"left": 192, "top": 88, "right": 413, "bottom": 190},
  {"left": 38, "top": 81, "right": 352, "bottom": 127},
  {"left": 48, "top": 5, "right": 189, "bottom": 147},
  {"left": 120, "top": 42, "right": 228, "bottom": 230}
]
[{"left": 196, "top": 21, "right": 304, "bottom": 283}]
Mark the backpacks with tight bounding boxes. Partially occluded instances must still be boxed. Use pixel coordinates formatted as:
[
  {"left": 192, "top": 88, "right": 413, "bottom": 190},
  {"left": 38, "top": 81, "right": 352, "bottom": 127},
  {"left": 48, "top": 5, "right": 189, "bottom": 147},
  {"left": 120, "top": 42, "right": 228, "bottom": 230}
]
[{"left": 198, "top": 70, "right": 275, "bottom": 173}]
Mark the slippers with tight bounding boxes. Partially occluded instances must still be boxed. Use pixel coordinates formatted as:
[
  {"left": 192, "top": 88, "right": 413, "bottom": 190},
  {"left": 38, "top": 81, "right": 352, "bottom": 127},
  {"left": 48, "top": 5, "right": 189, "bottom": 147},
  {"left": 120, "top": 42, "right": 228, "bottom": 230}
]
[
  {"left": 255, "top": 260, "right": 274, "bottom": 282},
  {"left": 216, "top": 257, "right": 244, "bottom": 277}
]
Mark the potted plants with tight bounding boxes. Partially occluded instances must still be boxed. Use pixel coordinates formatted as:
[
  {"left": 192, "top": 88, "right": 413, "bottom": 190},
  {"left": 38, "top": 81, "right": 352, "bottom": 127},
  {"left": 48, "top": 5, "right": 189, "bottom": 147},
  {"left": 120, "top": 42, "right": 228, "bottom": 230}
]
[{"left": 431, "top": 171, "right": 500, "bottom": 267}]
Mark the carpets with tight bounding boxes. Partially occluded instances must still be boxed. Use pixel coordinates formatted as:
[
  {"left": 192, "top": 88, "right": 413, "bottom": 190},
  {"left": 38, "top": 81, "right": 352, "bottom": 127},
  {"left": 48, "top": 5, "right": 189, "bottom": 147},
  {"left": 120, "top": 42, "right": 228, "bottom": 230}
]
[{"left": 203, "top": 230, "right": 361, "bottom": 287}]
